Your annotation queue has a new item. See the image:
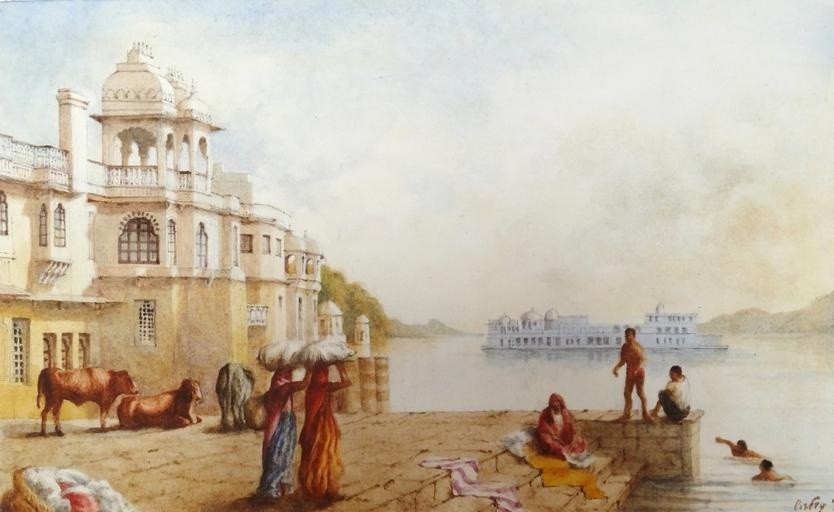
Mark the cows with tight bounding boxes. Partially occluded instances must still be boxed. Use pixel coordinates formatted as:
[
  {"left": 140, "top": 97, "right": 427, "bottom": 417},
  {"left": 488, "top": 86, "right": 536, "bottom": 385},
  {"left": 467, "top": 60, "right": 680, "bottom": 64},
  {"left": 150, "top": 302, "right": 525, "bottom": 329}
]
[
  {"left": 116, "top": 377, "right": 201, "bottom": 432},
  {"left": 215, "top": 361, "right": 255, "bottom": 431},
  {"left": 35, "top": 365, "right": 141, "bottom": 438}
]
